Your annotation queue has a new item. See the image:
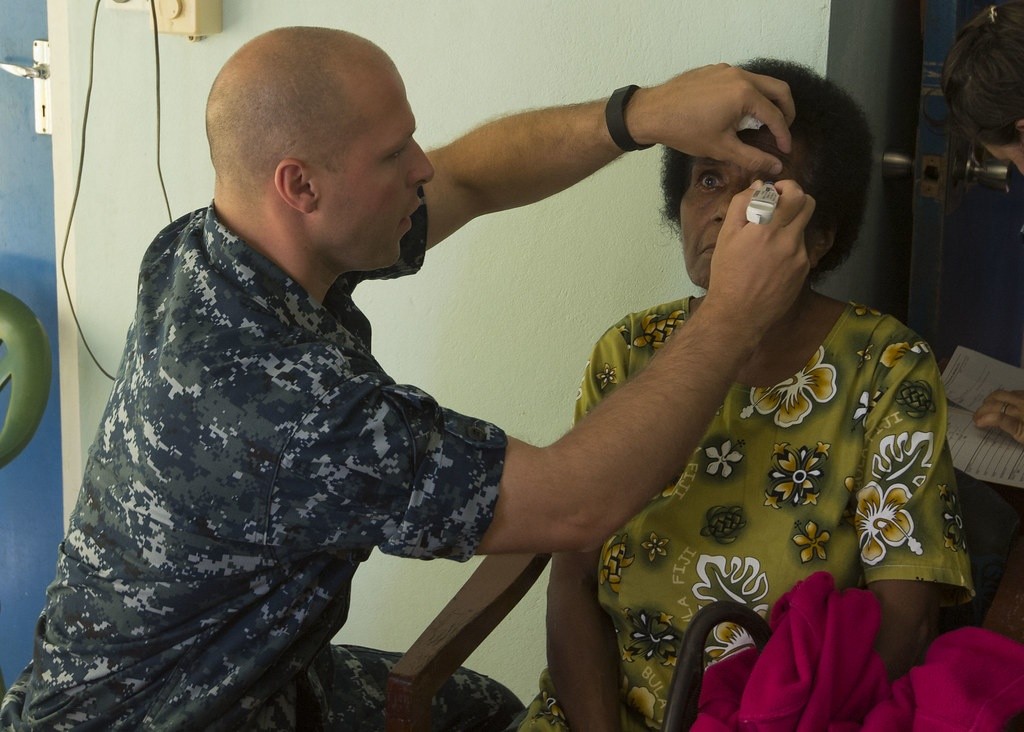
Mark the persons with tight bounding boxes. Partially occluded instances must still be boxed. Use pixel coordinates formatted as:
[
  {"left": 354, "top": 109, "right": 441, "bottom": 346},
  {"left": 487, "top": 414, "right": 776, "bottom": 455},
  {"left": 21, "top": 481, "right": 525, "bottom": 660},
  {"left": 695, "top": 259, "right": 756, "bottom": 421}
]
[
  {"left": 944, "top": 0, "right": 1024, "bottom": 652},
  {"left": 519, "top": 58, "right": 977, "bottom": 732},
  {"left": 0, "top": 26, "right": 816, "bottom": 732}
]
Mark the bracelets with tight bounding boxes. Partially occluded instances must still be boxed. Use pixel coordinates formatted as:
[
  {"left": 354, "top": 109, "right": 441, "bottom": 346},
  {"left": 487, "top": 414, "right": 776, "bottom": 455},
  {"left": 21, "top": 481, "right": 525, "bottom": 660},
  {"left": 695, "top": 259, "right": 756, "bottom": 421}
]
[{"left": 605, "top": 85, "right": 655, "bottom": 151}]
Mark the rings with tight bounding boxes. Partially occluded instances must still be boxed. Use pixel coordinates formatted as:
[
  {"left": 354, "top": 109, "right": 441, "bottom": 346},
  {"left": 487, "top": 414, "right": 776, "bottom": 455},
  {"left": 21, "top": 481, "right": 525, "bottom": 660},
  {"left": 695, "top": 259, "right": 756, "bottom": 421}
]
[{"left": 1000, "top": 403, "right": 1009, "bottom": 414}]
